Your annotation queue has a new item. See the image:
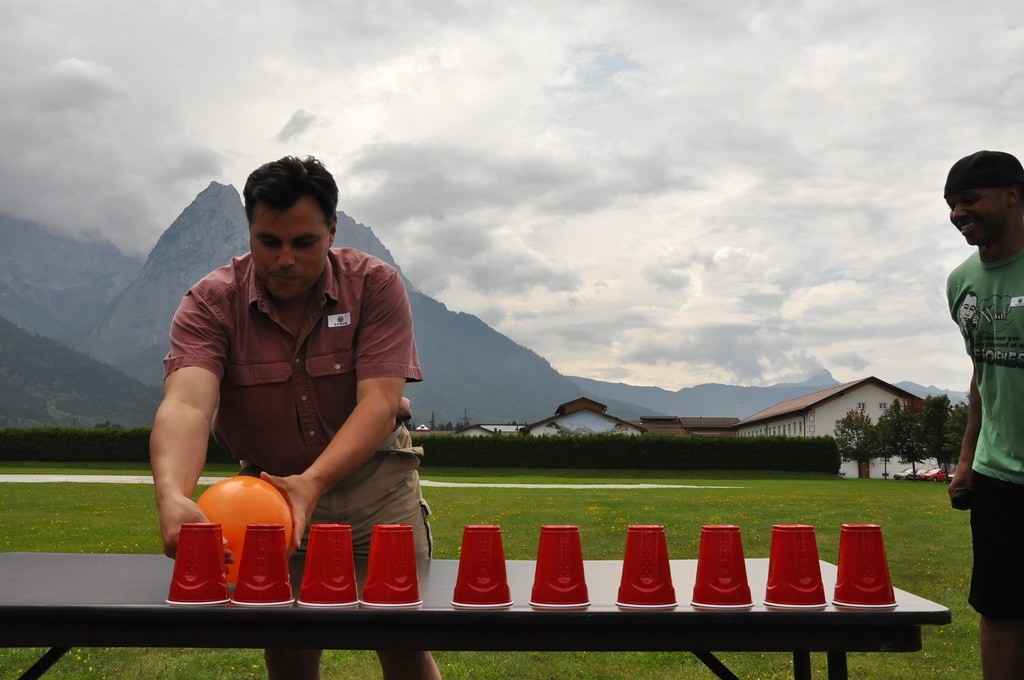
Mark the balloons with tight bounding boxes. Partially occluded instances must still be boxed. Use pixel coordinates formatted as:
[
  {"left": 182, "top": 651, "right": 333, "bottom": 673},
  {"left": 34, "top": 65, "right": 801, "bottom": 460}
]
[{"left": 193, "top": 476, "right": 294, "bottom": 582}]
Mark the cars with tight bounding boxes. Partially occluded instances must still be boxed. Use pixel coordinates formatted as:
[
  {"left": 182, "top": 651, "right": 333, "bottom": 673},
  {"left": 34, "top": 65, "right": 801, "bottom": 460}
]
[
  {"left": 922, "top": 467, "right": 955, "bottom": 482},
  {"left": 909, "top": 469, "right": 930, "bottom": 480},
  {"left": 893, "top": 468, "right": 918, "bottom": 480}
]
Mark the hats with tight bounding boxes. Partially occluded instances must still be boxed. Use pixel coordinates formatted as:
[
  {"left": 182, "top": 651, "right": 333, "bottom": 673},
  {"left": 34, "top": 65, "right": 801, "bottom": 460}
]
[{"left": 943, "top": 150, "right": 1024, "bottom": 198}]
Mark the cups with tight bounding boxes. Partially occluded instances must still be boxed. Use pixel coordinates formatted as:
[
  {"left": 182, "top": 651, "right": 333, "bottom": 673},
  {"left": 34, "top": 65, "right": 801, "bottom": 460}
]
[
  {"left": 231, "top": 520, "right": 296, "bottom": 606},
  {"left": 297, "top": 523, "right": 360, "bottom": 607},
  {"left": 762, "top": 523, "right": 829, "bottom": 609},
  {"left": 360, "top": 523, "right": 422, "bottom": 607},
  {"left": 165, "top": 522, "right": 230, "bottom": 605},
  {"left": 528, "top": 524, "right": 592, "bottom": 608},
  {"left": 614, "top": 525, "right": 679, "bottom": 608},
  {"left": 450, "top": 524, "right": 514, "bottom": 608},
  {"left": 832, "top": 523, "right": 898, "bottom": 608},
  {"left": 690, "top": 524, "right": 754, "bottom": 607}
]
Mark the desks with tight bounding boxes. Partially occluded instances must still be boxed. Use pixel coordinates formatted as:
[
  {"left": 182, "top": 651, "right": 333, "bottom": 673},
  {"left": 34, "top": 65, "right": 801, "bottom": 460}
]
[{"left": 1, "top": 554, "right": 952, "bottom": 680}]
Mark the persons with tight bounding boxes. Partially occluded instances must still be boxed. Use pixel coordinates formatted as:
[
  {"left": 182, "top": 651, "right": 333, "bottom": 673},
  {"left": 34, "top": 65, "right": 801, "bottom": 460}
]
[
  {"left": 943, "top": 149, "right": 1024, "bottom": 680},
  {"left": 148, "top": 153, "right": 441, "bottom": 680}
]
